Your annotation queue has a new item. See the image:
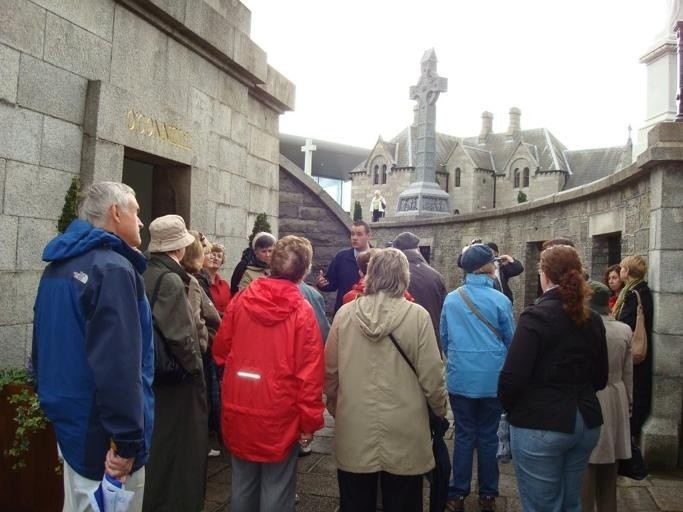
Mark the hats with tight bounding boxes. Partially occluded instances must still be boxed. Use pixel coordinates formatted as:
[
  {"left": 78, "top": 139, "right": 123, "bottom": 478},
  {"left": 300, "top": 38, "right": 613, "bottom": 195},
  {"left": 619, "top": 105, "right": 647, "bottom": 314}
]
[
  {"left": 147, "top": 214, "right": 195, "bottom": 253},
  {"left": 584, "top": 280, "right": 612, "bottom": 313},
  {"left": 461, "top": 244, "right": 504, "bottom": 273},
  {"left": 392, "top": 232, "right": 421, "bottom": 249}
]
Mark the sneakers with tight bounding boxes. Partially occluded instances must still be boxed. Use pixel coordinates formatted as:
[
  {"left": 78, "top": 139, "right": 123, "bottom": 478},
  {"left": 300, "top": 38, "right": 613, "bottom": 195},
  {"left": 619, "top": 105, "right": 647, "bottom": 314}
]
[
  {"left": 447, "top": 496, "right": 464, "bottom": 512},
  {"left": 477, "top": 495, "right": 496, "bottom": 512}
]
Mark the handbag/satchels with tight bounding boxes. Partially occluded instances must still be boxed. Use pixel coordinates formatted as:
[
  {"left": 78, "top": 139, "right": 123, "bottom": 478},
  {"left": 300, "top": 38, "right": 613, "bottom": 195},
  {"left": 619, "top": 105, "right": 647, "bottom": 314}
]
[
  {"left": 617, "top": 447, "right": 647, "bottom": 480},
  {"left": 151, "top": 321, "right": 186, "bottom": 385},
  {"left": 631, "top": 303, "right": 648, "bottom": 365}
]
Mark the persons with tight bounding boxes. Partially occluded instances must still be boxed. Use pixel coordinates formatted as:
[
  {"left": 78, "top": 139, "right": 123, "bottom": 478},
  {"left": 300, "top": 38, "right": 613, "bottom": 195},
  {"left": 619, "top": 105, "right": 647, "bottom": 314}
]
[
  {"left": 584, "top": 257, "right": 653, "bottom": 512},
  {"left": 32, "top": 181, "right": 156, "bottom": 512},
  {"left": 144, "top": 214, "right": 449, "bottom": 512},
  {"left": 369, "top": 190, "right": 386, "bottom": 222},
  {"left": 440, "top": 239, "right": 608, "bottom": 512}
]
